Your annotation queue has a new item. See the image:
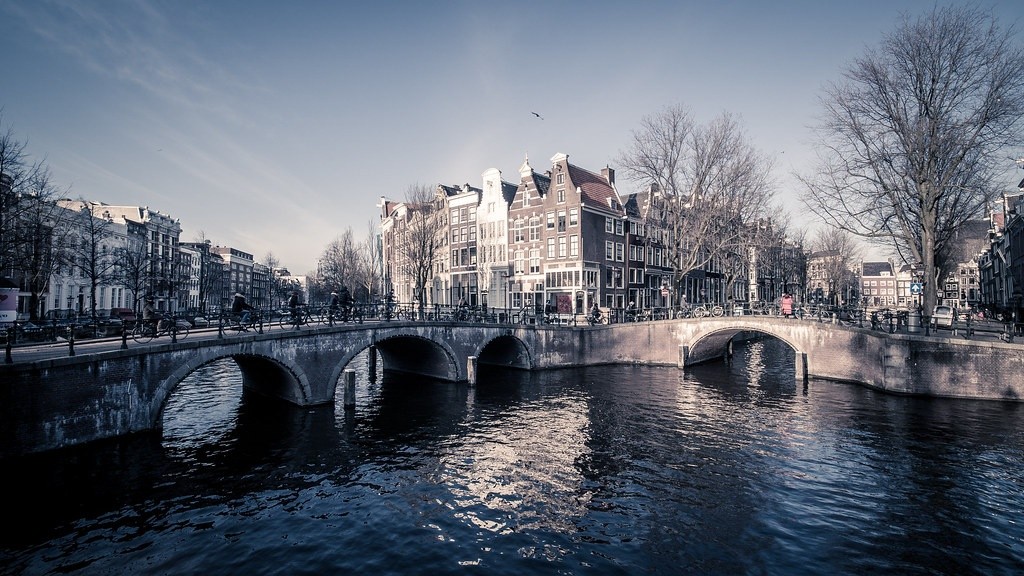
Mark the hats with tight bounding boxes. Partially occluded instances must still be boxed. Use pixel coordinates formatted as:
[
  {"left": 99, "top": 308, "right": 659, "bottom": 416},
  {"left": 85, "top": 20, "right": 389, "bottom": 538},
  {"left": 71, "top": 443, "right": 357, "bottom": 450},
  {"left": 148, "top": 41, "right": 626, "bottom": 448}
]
[
  {"left": 292, "top": 292, "right": 298, "bottom": 295},
  {"left": 682, "top": 295, "right": 687, "bottom": 298},
  {"left": 146, "top": 296, "right": 154, "bottom": 301}
]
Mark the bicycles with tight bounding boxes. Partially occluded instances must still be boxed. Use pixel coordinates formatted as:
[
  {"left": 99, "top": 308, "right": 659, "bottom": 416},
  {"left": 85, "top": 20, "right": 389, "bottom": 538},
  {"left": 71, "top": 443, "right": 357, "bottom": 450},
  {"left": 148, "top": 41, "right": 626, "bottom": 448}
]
[
  {"left": 279, "top": 304, "right": 321, "bottom": 331},
  {"left": 628, "top": 301, "right": 903, "bottom": 333},
  {"left": 321, "top": 302, "right": 366, "bottom": 325},
  {"left": 222, "top": 306, "right": 271, "bottom": 337},
  {"left": 375, "top": 304, "right": 411, "bottom": 321},
  {"left": 131, "top": 311, "right": 189, "bottom": 344}
]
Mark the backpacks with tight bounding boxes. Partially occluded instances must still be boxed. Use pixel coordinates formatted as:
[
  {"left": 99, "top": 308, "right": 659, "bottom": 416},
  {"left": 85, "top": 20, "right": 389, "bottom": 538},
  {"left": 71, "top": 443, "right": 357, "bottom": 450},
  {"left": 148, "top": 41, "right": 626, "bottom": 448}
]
[{"left": 383, "top": 294, "right": 387, "bottom": 299}]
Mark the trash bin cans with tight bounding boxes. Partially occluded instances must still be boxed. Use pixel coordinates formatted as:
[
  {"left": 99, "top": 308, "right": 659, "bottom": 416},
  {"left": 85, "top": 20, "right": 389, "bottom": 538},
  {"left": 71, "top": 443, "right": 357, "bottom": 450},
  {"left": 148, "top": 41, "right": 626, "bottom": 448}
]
[{"left": 908, "top": 313, "right": 921, "bottom": 334}]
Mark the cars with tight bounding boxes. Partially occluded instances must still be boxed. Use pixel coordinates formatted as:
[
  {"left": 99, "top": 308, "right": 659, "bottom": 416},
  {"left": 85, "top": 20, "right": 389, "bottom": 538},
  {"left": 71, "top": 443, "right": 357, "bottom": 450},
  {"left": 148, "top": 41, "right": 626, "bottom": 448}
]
[{"left": 41, "top": 308, "right": 134, "bottom": 339}]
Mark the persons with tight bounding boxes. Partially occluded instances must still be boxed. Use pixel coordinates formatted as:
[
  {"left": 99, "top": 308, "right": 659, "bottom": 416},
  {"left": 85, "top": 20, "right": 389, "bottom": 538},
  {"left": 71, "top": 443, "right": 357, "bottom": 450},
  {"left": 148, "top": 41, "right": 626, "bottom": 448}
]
[
  {"left": 456, "top": 296, "right": 468, "bottom": 312},
  {"left": 545, "top": 300, "right": 551, "bottom": 315},
  {"left": 780, "top": 293, "right": 792, "bottom": 319},
  {"left": 288, "top": 291, "right": 304, "bottom": 318},
  {"left": 412, "top": 296, "right": 420, "bottom": 320},
  {"left": 591, "top": 303, "right": 603, "bottom": 326},
  {"left": 329, "top": 286, "right": 356, "bottom": 314},
  {"left": 142, "top": 296, "right": 163, "bottom": 334},
  {"left": 680, "top": 294, "right": 689, "bottom": 318},
  {"left": 232, "top": 291, "right": 254, "bottom": 324},
  {"left": 386, "top": 289, "right": 395, "bottom": 302}
]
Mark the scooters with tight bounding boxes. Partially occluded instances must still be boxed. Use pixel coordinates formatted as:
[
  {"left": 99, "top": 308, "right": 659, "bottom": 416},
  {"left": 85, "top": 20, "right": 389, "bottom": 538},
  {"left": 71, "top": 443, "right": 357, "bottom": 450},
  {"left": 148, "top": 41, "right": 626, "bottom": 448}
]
[{"left": 585, "top": 312, "right": 609, "bottom": 326}]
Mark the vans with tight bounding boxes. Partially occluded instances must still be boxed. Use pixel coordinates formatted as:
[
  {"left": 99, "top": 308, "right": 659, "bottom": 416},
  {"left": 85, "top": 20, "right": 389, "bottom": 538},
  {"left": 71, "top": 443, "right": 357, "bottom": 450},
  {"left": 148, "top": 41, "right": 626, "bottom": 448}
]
[{"left": 932, "top": 305, "right": 958, "bottom": 327}]
